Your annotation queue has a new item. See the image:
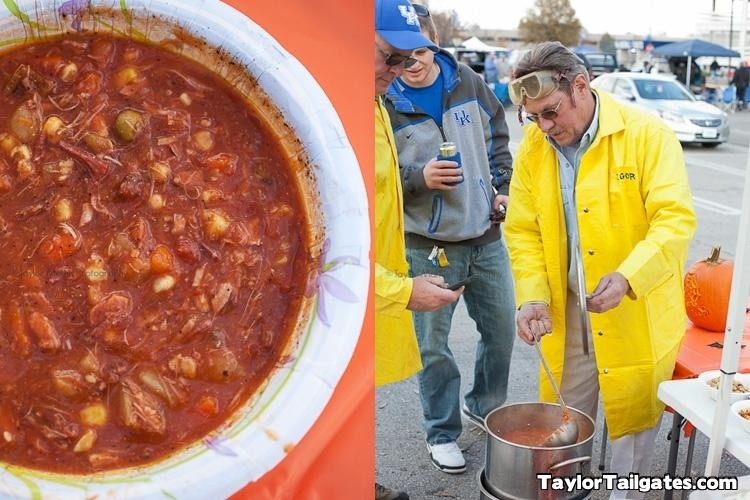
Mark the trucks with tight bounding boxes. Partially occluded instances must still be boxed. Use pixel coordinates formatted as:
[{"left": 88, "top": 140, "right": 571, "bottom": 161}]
[{"left": 453, "top": 46, "right": 513, "bottom": 105}]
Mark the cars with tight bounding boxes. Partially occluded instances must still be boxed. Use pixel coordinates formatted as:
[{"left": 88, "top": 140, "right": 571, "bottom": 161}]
[{"left": 588, "top": 72, "right": 730, "bottom": 149}]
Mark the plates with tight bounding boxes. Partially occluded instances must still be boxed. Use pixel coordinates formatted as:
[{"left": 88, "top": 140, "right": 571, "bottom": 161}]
[{"left": 0, "top": 1, "right": 370, "bottom": 500}]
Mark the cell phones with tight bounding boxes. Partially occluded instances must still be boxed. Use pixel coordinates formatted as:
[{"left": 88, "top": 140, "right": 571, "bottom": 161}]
[
  {"left": 488, "top": 209, "right": 505, "bottom": 221},
  {"left": 444, "top": 270, "right": 467, "bottom": 289}
]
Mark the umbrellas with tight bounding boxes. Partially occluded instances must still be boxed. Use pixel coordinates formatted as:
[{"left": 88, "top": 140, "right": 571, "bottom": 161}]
[{"left": 649, "top": 34, "right": 742, "bottom": 90}]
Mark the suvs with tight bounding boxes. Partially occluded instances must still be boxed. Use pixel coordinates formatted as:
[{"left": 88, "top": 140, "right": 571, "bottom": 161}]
[{"left": 584, "top": 52, "right": 618, "bottom": 77}]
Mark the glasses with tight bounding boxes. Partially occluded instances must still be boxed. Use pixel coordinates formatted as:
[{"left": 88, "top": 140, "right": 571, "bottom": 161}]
[
  {"left": 526, "top": 83, "right": 573, "bottom": 124},
  {"left": 375, "top": 44, "right": 419, "bottom": 70},
  {"left": 507, "top": 69, "right": 561, "bottom": 106},
  {"left": 411, "top": 5, "right": 433, "bottom": 17}
]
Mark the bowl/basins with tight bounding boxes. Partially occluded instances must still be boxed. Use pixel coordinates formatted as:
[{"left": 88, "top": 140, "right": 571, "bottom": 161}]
[
  {"left": 698, "top": 370, "right": 750, "bottom": 404},
  {"left": 731, "top": 401, "right": 750, "bottom": 434}
]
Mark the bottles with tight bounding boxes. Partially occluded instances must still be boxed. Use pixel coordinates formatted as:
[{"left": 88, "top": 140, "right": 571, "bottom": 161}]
[{"left": 437, "top": 141, "right": 464, "bottom": 186}]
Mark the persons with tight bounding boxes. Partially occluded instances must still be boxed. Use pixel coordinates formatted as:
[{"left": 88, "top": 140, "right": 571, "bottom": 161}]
[
  {"left": 374, "top": 1, "right": 466, "bottom": 500},
  {"left": 383, "top": 4, "right": 519, "bottom": 475},
  {"left": 500, "top": 40, "right": 698, "bottom": 499},
  {"left": 454, "top": 59, "right": 750, "bottom": 113}
]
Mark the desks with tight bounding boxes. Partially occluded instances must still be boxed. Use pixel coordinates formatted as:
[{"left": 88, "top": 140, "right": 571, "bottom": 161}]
[{"left": 663, "top": 302, "right": 750, "bottom": 500}]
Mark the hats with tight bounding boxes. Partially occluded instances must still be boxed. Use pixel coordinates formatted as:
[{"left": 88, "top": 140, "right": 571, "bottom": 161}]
[{"left": 374, "top": 1, "right": 442, "bottom": 55}]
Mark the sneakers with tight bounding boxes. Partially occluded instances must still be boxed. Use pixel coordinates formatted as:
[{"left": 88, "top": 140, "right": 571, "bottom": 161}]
[
  {"left": 375, "top": 482, "right": 408, "bottom": 498},
  {"left": 426, "top": 438, "right": 466, "bottom": 473},
  {"left": 462, "top": 402, "right": 485, "bottom": 431}
]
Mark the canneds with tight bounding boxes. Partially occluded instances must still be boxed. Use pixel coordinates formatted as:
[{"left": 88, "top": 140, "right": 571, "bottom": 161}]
[{"left": 440, "top": 143, "right": 456, "bottom": 156}]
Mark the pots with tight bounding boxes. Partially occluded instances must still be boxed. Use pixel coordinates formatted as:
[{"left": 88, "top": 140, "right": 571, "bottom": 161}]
[{"left": 457, "top": 398, "right": 598, "bottom": 500}]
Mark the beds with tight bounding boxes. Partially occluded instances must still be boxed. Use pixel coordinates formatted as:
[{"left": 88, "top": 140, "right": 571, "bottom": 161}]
[{"left": 657, "top": 373, "right": 750, "bottom": 500}]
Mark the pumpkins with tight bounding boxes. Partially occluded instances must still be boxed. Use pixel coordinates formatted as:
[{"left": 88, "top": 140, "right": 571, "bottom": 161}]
[{"left": 685, "top": 246, "right": 735, "bottom": 331}]
